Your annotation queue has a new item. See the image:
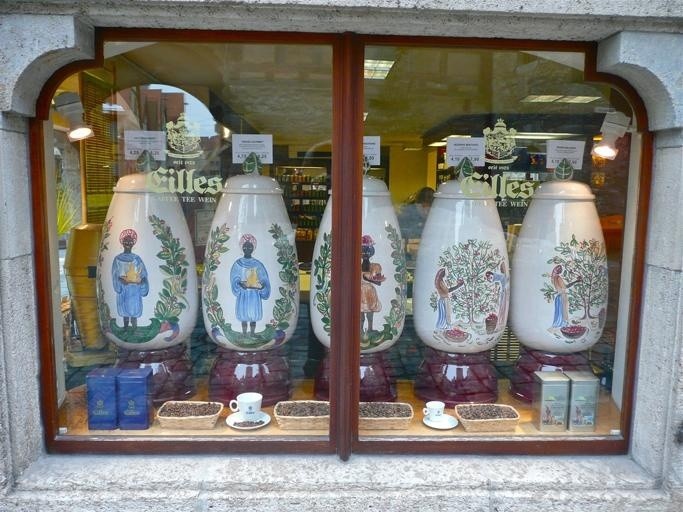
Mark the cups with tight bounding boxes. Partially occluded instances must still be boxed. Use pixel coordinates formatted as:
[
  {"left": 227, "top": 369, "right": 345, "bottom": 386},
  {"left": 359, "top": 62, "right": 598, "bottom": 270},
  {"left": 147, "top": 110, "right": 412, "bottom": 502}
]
[
  {"left": 228, "top": 392, "right": 262, "bottom": 416},
  {"left": 423, "top": 401, "right": 446, "bottom": 419}
]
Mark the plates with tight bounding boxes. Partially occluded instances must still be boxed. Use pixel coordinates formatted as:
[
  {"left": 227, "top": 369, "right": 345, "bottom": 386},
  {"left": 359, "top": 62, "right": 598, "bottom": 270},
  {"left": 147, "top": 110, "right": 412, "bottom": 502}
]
[
  {"left": 224, "top": 412, "right": 273, "bottom": 428},
  {"left": 424, "top": 416, "right": 461, "bottom": 429}
]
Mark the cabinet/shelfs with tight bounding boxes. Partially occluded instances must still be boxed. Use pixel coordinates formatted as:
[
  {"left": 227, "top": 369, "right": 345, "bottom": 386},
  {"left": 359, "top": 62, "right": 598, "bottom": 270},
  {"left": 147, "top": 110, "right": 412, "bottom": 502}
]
[{"left": 275, "top": 166, "right": 328, "bottom": 229}]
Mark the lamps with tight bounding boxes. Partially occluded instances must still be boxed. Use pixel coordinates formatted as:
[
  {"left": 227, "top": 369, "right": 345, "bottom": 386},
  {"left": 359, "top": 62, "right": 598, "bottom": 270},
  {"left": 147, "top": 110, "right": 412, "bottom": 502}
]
[
  {"left": 53, "top": 91, "right": 96, "bottom": 144},
  {"left": 517, "top": 78, "right": 605, "bottom": 104},
  {"left": 100, "top": 85, "right": 133, "bottom": 115},
  {"left": 591, "top": 109, "right": 631, "bottom": 160},
  {"left": 363, "top": 51, "right": 402, "bottom": 81}
]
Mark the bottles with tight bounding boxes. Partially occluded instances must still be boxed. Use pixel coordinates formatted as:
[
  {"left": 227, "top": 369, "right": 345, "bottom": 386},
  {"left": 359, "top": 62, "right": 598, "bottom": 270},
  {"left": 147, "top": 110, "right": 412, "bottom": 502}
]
[{"left": 65, "top": 172, "right": 609, "bottom": 406}]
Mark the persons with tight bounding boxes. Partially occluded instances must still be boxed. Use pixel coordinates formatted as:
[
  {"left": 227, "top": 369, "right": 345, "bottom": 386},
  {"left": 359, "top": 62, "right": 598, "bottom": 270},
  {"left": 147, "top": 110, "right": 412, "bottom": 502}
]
[
  {"left": 113, "top": 229, "right": 149, "bottom": 337},
  {"left": 398, "top": 187, "right": 435, "bottom": 239},
  {"left": 486, "top": 262, "right": 507, "bottom": 326},
  {"left": 551, "top": 265, "right": 582, "bottom": 327},
  {"left": 360, "top": 246, "right": 381, "bottom": 335},
  {"left": 434, "top": 269, "right": 464, "bottom": 331},
  {"left": 231, "top": 234, "right": 270, "bottom": 337}
]
